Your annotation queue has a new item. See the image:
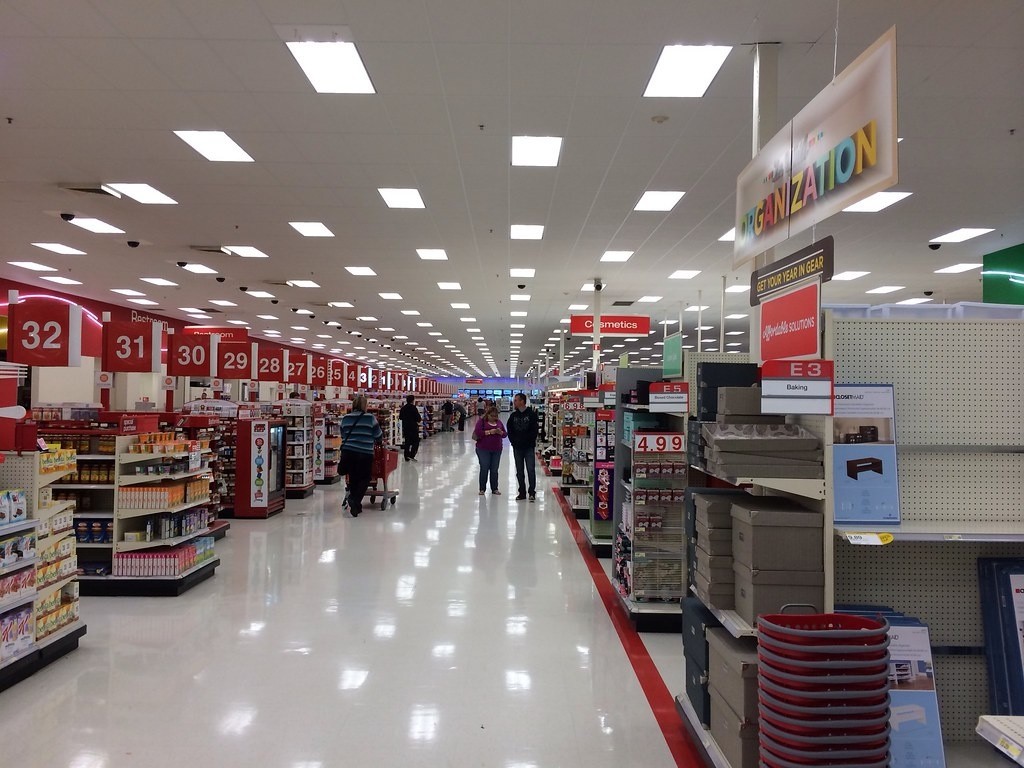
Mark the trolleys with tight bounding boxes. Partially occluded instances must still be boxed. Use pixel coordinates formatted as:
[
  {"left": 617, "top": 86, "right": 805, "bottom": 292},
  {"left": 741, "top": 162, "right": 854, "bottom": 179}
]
[
  {"left": 756, "top": 603, "right": 892, "bottom": 768},
  {"left": 342, "top": 437, "right": 399, "bottom": 510}
]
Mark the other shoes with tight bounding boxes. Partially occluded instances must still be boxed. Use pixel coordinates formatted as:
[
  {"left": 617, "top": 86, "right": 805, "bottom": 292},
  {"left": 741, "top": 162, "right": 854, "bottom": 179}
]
[
  {"left": 351, "top": 504, "right": 362, "bottom": 517},
  {"left": 516, "top": 493, "right": 535, "bottom": 500},
  {"left": 407, "top": 455, "right": 417, "bottom": 462},
  {"left": 492, "top": 490, "right": 501, "bottom": 495},
  {"left": 479, "top": 490, "right": 485, "bottom": 495}
]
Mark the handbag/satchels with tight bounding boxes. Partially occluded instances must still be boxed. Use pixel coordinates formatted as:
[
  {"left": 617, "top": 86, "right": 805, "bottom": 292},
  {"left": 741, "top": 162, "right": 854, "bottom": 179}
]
[
  {"left": 472, "top": 430, "right": 480, "bottom": 441},
  {"left": 337, "top": 449, "right": 349, "bottom": 476}
]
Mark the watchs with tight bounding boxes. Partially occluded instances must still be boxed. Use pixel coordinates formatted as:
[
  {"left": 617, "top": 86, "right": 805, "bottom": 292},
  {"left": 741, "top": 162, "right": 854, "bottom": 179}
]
[{"left": 487, "top": 430, "right": 490, "bottom": 435}]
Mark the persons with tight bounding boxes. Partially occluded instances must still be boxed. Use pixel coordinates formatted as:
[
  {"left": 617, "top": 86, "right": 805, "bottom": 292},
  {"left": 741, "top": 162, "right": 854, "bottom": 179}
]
[
  {"left": 441, "top": 398, "right": 453, "bottom": 432},
  {"left": 477, "top": 397, "right": 486, "bottom": 419},
  {"left": 507, "top": 393, "right": 539, "bottom": 501},
  {"left": 340, "top": 396, "right": 382, "bottom": 517},
  {"left": 452, "top": 401, "right": 466, "bottom": 433},
  {"left": 399, "top": 395, "right": 421, "bottom": 462},
  {"left": 289, "top": 392, "right": 300, "bottom": 399},
  {"left": 474, "top": 407, "right": 508, "bottom": 495}
]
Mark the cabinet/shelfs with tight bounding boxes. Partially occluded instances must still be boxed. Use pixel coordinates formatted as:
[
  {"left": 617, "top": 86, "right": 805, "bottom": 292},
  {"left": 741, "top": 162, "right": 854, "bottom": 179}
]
[
  {"left": 675, "top": 298, "right": 1024, "bottom": 768},
  {"left": 529, "top": 368, "right": 684, "bottom": 633},
  {"left": 35, "top": 416, "right": 220, "bottom": 597},
  {"left": 98, "top": 401, "right": 476, "bottom": 639},
  {"left": 0, "top": 450, "right": 87, "bottom": 693}
]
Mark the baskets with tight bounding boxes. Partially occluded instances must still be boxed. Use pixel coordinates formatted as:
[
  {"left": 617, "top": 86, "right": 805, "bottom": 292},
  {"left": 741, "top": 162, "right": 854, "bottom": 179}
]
[{"left": 754, "top": 612, "right": 893, "bottom": 768}]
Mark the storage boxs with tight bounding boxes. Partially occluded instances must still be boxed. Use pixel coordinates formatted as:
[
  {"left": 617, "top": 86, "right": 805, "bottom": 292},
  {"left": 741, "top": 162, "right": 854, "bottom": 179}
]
[
  {"left": 114, "top": 479, "right": 215, "bottom": 576},
  {"left": 682, "top": 362, "right": 825, "bottom": 768},
  {"left": 624, "top": 411, "right": 656, "bottom": 430},
  {"left": 834, "top": 604, "right": 947, "bottom": 768},
  {"left": 624, "top": 430, "right": 630, "bottom": 441},
  {"left": 0, "top": 488, "right": 79, "bottom": 663},
  {"left": 0, "top": 361, "right": 28, "bottom": 450},
  {"left": 630, "top": 421, "right": 659, "bottom": 440}
]
